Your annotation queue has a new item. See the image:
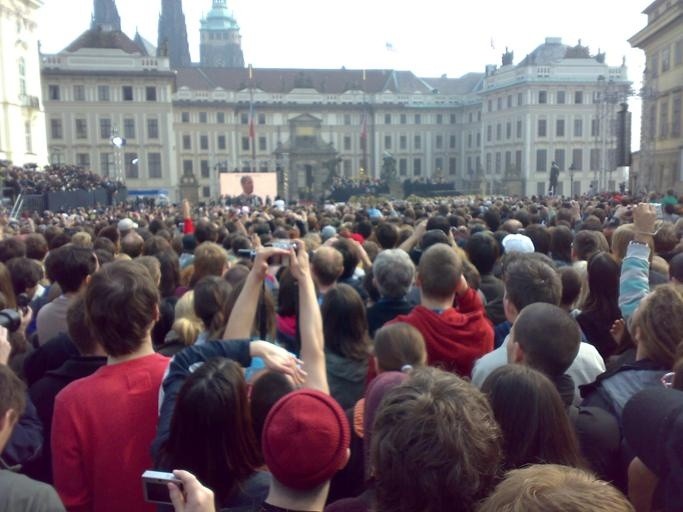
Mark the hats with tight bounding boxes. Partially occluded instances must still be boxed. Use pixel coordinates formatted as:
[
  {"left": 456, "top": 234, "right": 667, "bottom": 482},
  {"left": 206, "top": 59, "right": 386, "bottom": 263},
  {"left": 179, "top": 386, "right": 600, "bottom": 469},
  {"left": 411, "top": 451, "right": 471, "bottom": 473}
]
[
  {"left": 262, "top": 390, "right": 352, "bottom": 493},
  {"left": 118, "top": 218, "right": 139, "bottom": 231},
  {"left": 501, "top": 234, "right": 535, "bottom": 256},
  {"left": 321, "top": 225, "right": 340, "bottom": 237},
  {"left": 622, "top": 388, "right": 682, "bottom": 496}
]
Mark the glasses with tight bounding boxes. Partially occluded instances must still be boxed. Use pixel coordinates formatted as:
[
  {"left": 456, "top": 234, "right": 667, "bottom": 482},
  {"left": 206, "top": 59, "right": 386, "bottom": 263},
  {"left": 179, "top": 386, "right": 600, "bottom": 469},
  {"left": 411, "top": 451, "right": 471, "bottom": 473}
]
[{"left": 660, "top": 372, "right": 676, "bottom": 388}]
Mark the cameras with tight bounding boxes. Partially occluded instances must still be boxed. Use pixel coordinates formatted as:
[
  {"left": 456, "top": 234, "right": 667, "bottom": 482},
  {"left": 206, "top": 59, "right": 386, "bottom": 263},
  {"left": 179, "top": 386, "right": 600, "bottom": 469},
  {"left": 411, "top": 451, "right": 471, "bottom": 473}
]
[
  {"left": 0, "top": 308, "right": 20, "bottom": 330},
  {"left": 262, "top": 241, "right": 297, "bottom": 267},
  {"left": 648, "top": 203, "right": 663, "bottom": 219},
  {"left": 140, "top": 470, "right": 185, "bottom": 506}
]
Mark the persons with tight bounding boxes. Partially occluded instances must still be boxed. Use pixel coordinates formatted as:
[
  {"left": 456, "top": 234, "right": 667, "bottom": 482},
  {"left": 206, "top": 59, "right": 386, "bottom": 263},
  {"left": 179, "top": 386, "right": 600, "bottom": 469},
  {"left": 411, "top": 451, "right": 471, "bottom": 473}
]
[{"left": 2, "top": 162, "right": 682, "bottom": 510}]
